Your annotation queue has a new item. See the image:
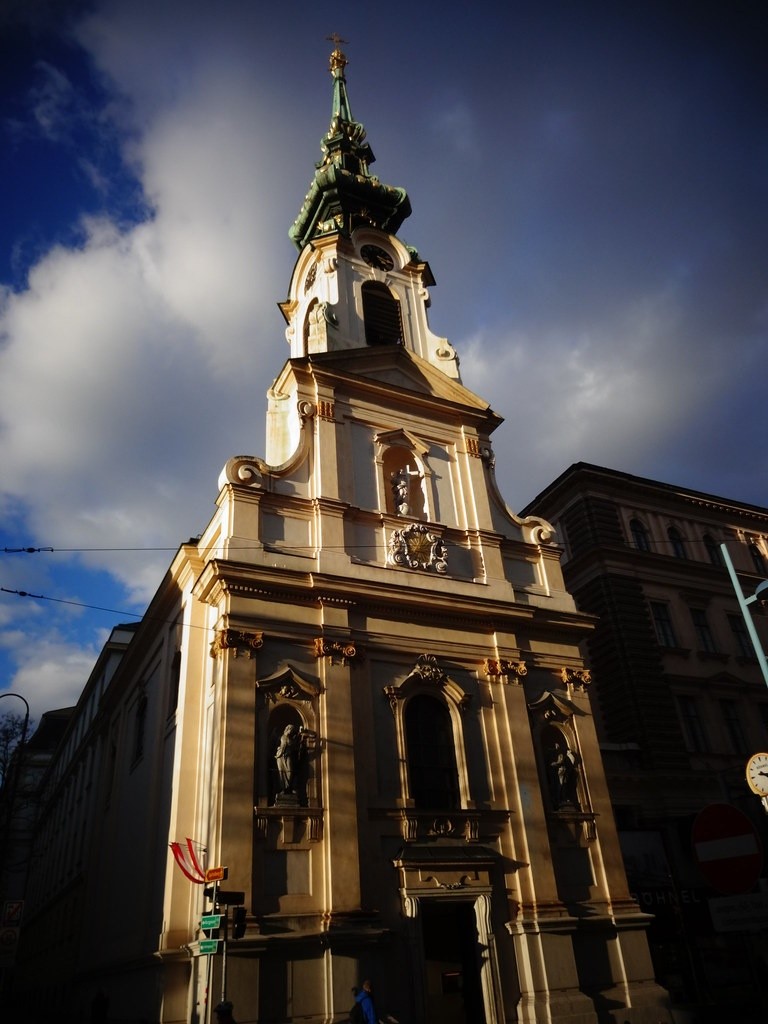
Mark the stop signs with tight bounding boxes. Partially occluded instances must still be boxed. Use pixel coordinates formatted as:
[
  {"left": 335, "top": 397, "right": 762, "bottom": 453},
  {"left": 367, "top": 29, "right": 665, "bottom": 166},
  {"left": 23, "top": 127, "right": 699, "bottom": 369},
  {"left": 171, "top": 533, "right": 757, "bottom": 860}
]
[{"left": 691, "top": 801, "right": 763, "bottom": 895}]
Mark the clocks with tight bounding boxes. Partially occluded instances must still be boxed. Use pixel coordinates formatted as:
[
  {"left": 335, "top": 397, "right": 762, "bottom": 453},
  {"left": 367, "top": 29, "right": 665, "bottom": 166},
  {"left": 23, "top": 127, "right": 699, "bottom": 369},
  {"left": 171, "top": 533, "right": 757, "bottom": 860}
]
[
  {"left": 360, "top": 244, "right": 395, "bottom": 272},
  {"left": 746, "top": 752, "right": 768, "bottom": 798}
]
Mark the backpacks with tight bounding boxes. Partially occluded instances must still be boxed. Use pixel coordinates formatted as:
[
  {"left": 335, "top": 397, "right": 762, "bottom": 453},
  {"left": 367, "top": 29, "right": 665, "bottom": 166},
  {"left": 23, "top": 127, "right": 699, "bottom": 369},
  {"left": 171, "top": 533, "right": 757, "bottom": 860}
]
[{"left": 351, "top": 995, "right": 372, "bottom": 1024}]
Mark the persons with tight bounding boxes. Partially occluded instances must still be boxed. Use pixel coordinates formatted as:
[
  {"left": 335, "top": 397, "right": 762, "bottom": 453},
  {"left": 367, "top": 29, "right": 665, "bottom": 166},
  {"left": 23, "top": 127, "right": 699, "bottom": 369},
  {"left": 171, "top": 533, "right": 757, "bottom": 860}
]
[
  {"left": 550, "top": 743, "right": 567, "bottom": 784},
  {"left": 390, "top": 469, "right": 409, "bottom": 515},
  {"left": 274, "top": 724, "right": 296, "bottom": 794},
  {"left": 354, "top": 979, "right": 377, "bottom": 1024}
]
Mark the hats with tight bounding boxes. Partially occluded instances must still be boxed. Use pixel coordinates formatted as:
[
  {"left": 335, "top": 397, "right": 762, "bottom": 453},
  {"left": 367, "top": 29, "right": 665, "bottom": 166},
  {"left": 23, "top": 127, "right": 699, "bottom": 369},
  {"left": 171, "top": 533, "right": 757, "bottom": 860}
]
[{"left": 213, "top": 1001, "right": 233, "bottom": 1012}]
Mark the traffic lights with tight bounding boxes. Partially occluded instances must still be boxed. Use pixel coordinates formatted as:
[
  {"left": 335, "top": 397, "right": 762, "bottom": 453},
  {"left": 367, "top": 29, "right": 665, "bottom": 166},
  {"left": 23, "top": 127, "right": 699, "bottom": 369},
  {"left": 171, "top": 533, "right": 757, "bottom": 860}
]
[
  {"left": 203, "top": 885, "right": 219, "bottom": 902},
  {"left": 199, "top": 908, "right": 220, "bottom": 939},
  {"left": 232, "top": 907, "right": 248, "bottom": 939}
]
